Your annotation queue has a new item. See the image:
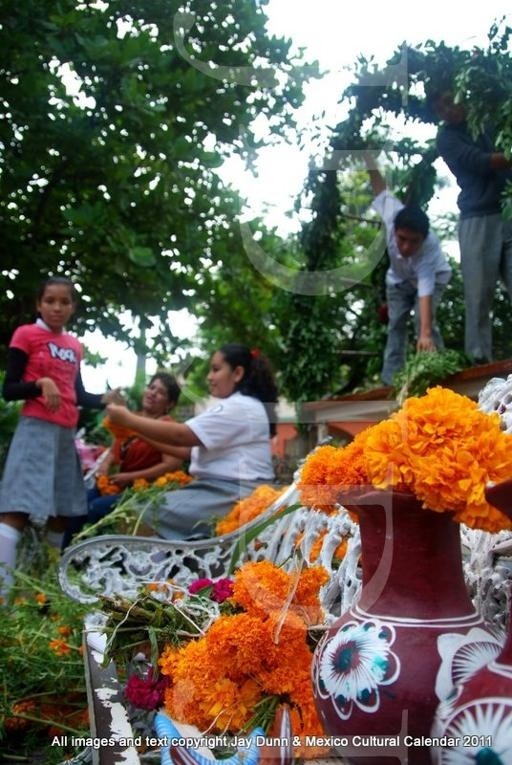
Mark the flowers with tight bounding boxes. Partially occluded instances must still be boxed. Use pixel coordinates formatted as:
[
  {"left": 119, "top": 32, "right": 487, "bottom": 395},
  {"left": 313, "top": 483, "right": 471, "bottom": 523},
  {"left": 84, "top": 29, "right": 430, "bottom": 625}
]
[{"left": 94, "top": 388, "right": 509, "bottom": 765}]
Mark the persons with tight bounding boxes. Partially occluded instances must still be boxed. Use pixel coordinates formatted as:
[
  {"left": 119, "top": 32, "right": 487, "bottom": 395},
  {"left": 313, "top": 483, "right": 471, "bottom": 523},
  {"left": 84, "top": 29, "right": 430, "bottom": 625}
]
[
  {"left": 362, "top": 150, "right": 452, "bottom": 386},
  {"left": 429, "top": 87, "right": 511, "bottom": 366},
  {"left": 106, "top": 343, "right": 279, "bottom": 545},
  {"left": 1, "top": 276, "right": 125, "bottom": 610},
  {"left": 60, "top": 371, "right": 184, "bottom": 554}
]
